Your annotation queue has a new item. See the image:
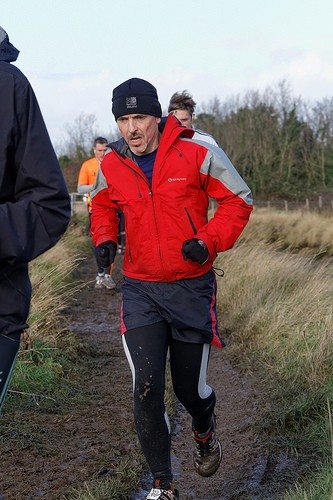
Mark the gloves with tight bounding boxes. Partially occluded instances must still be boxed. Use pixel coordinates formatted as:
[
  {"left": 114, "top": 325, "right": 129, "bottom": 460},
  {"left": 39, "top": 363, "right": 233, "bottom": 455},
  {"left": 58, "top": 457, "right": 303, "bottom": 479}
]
[
  {"left": 181, "top": 237, "right": 209, "bottom": 266},
  {"left": 95, "top": 241, "right": 118, "bottom": 268}
]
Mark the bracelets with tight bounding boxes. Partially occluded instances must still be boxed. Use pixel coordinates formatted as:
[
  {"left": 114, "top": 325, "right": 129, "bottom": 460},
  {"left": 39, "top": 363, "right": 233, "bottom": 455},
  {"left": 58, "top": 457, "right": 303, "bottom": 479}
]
[{"left": 199, "top": 238, "right": 206, "bottom": 251}]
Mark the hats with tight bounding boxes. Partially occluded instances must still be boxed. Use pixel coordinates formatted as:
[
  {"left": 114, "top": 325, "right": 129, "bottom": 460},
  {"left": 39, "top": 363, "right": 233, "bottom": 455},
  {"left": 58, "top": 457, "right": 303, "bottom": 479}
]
[{"left": 111, "top": 77, "right": 162, "bottom": 121}]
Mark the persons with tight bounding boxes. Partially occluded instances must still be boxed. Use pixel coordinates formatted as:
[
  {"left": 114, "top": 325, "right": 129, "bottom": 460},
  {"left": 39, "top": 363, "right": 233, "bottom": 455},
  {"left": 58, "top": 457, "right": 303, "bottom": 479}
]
[
  {"left": 78, "top": 136, "right": 117, "bottom": 290},
  {"left": 91, "top": 78, "right": 254, "bottom": 500},
  {"left": 167, "top": 92, "right": 196, "bottom": 131},
  {"left": 0, "top": 23, "right": 70, "bottom": 409},
  {"left": 117, "top": 207, "right": 126, "bottom": 255}
]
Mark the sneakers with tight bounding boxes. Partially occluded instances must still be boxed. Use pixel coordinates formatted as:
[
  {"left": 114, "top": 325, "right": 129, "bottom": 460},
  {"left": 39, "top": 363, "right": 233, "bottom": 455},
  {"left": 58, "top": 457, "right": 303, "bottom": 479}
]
[
  {"left": 102, "top": 274, "right": 116, "bottom": 289},
  {"left": 143, "top": 479, "right": 178, "bottom": 500},
  {"left": 94, "top": 272, "right": 105, "bottom": 289},
  {"left": 191, "top": 413, "right": 222, "bottom": 477}
]
